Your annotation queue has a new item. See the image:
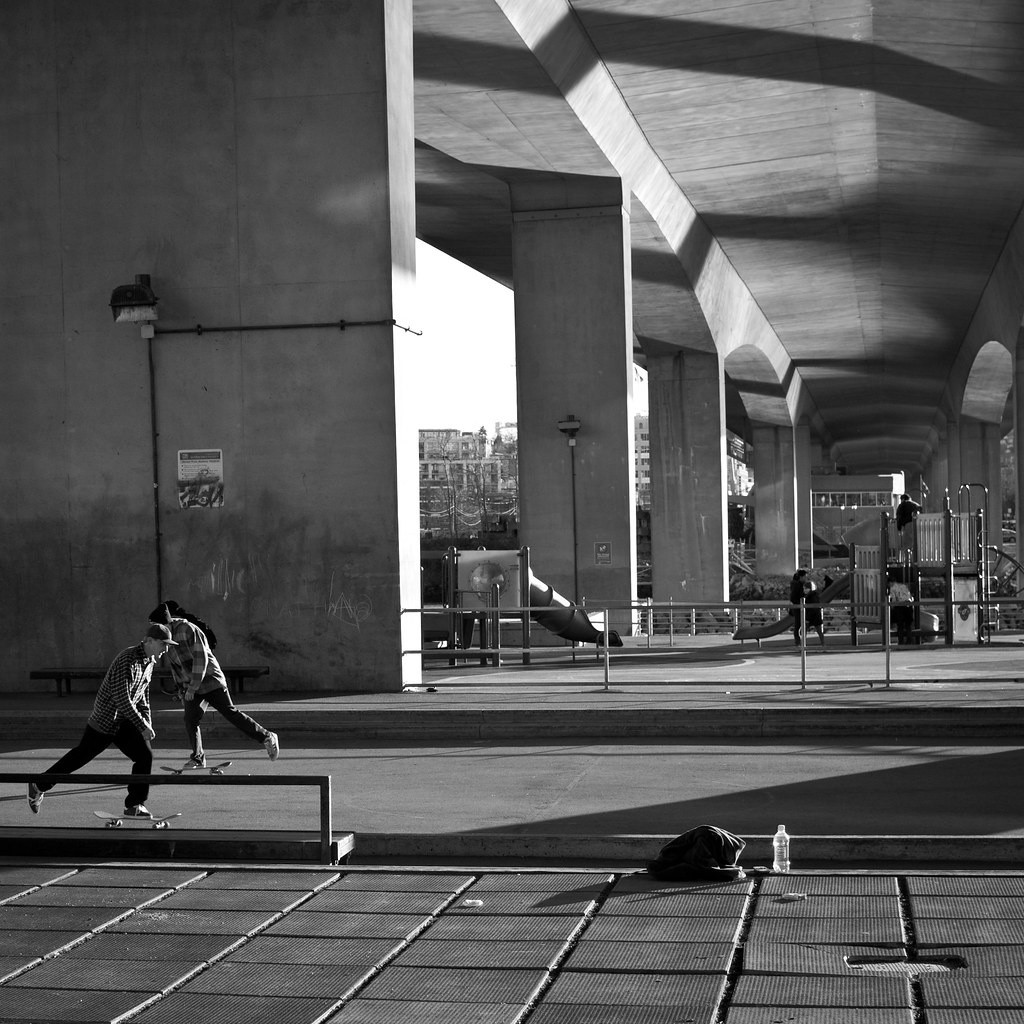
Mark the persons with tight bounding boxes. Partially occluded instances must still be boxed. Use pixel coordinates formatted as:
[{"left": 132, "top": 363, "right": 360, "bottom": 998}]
[
  {"left": 26, "top": 623, "right": 179, "bottom": 819},
  {"left": 148, "top": 603, "right": 280, "bottom": 769},
  {"left": 788, "top": 493, "right": 928, "bottom": 647}
]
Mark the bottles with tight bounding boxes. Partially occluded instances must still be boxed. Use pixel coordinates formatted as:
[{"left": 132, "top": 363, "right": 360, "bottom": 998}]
[{"left": 773, "top": 825, "right": 789, "bottom": 872}]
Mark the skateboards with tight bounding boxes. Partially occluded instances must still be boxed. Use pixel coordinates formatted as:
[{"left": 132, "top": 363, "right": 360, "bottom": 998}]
[
  {"left": 160, "top": 761, "right": 235, "bottom": 776},
  {"left": 94, "top": 809, "right": 185, "bottom": 829}
]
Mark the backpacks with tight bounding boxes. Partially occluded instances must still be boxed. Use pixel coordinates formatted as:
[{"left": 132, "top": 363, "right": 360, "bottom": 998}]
[{"left": 172, "top": 613, "right": 217, "bottom": 649}]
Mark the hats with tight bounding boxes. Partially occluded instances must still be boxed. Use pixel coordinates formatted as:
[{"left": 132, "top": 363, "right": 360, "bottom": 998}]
[
  {"left": 148, "top": 603, "right": 172, "bottom": 624},
  {"left": 147, "top": 624, "right": 179, "bottom": 645}
]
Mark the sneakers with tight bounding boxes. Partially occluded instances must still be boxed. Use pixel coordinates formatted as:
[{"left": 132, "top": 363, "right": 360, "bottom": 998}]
[
  {"left": 183, "top": 759, "right": 206, "bottom": 770},
  {"left": 28, "top": 780, "right": 45, "bottom": 814},
  {"left": 123, "top": 804, "right": 153, "bottom": 819},
  {"left": 264, "top": 731, "right": 279, "bottom": 761}
]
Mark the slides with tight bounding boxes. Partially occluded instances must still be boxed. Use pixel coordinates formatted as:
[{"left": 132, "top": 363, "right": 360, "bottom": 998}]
[
  {"left": 730, "top": 570, "right": 853, "bottom": 639},
  {"left": 529, "top": 570, "right": 625, "bottom": 649}
]
